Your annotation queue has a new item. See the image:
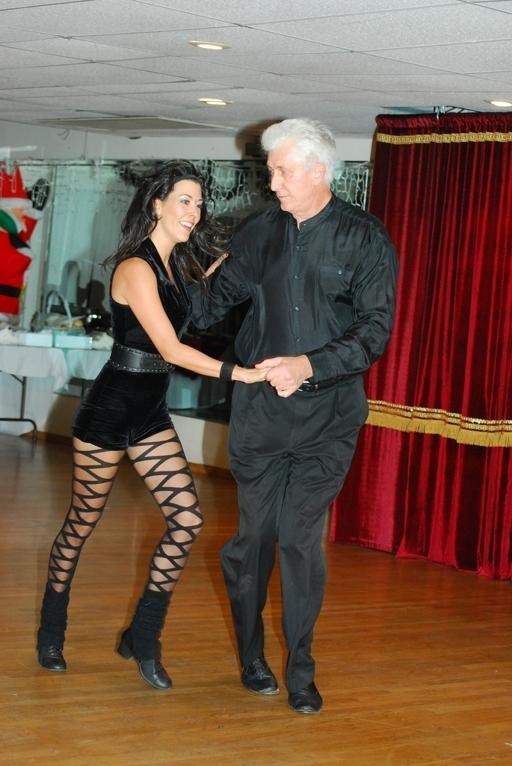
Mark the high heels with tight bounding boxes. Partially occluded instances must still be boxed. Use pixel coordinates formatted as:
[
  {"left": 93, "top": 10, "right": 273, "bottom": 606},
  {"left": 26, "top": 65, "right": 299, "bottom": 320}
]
[
  {"left": 37, "top": 645, "right": 67, "bottom": 672},
  {"left": 117, "top": 627, "right": 172, "bottom": 689}
]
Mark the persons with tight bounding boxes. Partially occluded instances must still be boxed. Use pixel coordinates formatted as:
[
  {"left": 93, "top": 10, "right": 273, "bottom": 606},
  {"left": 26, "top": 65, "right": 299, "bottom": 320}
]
[
  {"left": 36, "top": 158, "right": 274, "bottom": 691},
  {"left": 186, "top": 118, "right": 400, "bottom": 714}
]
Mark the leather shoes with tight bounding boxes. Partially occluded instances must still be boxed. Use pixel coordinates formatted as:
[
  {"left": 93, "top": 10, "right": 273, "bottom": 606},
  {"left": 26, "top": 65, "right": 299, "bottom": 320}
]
[
  {"left": 241, "top": 656, "right": 281, "bottom": 695},
  {"left": 288, "top": 680, "right": 323, "bottom": 714}
]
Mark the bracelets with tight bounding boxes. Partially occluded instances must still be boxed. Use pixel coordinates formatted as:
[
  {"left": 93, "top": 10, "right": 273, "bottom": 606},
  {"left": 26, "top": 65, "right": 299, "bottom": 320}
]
[{"left": 220, "top": 360, "right": 237, "bottom": 383}]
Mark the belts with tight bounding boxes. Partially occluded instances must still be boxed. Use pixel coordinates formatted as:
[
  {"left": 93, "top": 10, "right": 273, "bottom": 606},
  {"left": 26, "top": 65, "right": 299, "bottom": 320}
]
[
  {"left": 297, "top": 378, "right": 340, "bottom": 392},
  {"left": 109, "top": 342, "right": 176, "bottom": 374}
]
